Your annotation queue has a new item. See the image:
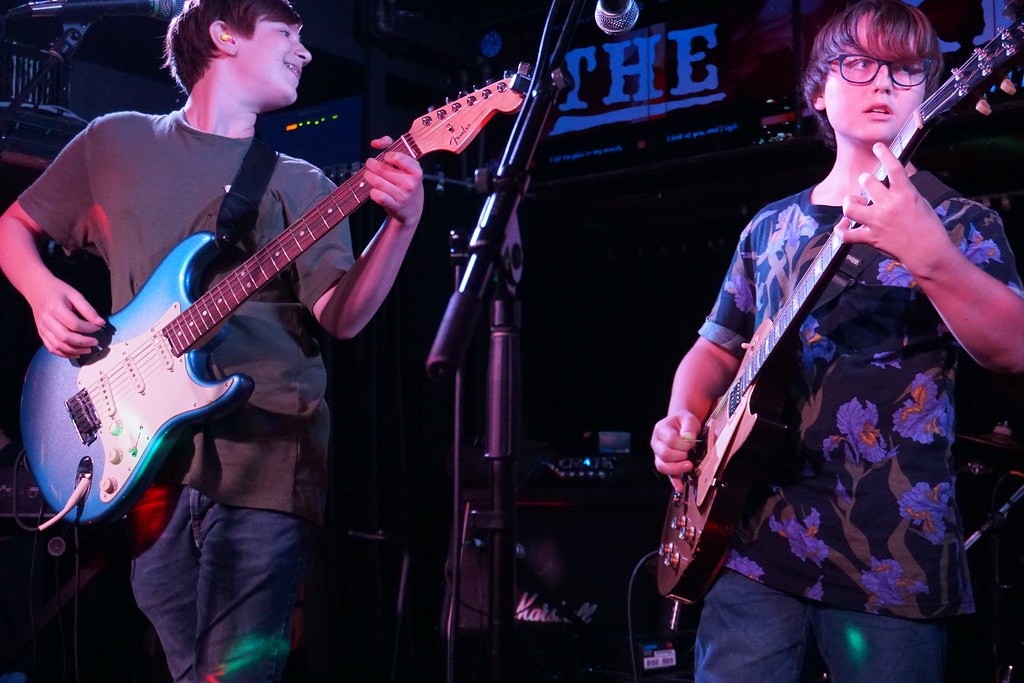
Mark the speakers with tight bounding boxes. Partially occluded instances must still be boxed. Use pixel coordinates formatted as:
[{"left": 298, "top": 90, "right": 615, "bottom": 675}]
[
  {"left": 442, "top": 488, "right": 704, "bottom": 683},
  {"left": 0, "top": 512, "right": 146, "bottom": 683}
]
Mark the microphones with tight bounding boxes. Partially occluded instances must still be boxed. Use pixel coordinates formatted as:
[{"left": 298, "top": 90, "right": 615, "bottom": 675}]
[
  {"left": 0, "top": 0, "right": 184, "bottom": 22},
  {"left": 595, "top": 0, "right": 640, "bottom": 36}
]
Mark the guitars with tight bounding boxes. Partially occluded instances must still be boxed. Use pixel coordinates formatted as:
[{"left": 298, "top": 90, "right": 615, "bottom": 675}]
[
  {"left": 18, "top": 60, "right": 532, "bottom": 532},
  {"left": 652, "top": 13, "right": 1024, "bottom": 609}
]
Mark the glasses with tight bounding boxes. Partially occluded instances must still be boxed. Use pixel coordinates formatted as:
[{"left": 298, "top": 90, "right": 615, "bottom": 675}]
[{"left": 828, "top": 54, "right": 932, "bottom": 87}]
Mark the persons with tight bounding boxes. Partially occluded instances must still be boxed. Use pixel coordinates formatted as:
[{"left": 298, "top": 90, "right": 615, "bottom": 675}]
[
  {"left": 0, "top": 0, "right": 425, "bottom": 683},
  {"left": 650, "top": 0, "right": 1024, "bottom": 683}
]
